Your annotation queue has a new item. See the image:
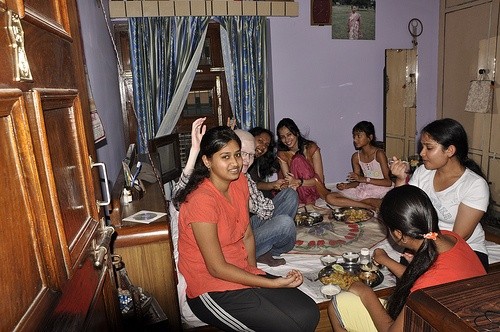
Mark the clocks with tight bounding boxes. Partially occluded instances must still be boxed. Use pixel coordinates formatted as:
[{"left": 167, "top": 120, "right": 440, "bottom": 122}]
[{"left": 310, "top": 0, "right": 332, "bottom": 26}]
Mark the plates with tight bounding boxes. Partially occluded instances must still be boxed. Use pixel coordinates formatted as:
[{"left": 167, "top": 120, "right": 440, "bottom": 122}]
[
  {"left": 320, "top": 285, "right": 341, "bottom": 296},
  {"left": 318, "top": 262, "right": 384, "bottom": 288},
  {"left": 294, "top": 212, "right": 323, "bottom": 227},
  {"left": 332, "top": 206, "right": 375, "bottom": 224}
]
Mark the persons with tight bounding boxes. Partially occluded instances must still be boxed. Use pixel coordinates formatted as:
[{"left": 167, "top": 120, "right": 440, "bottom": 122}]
[
  {"left": 346, "top": 3, "right": 361, "bottom": 39},
  {"left": 177, "top": 126, "right": 320, "bottom": 332},
  {"left": 390, "top": 118, "right": 490, "bottom": 285},
  {"left": 326, "top": 185, "right": 488, "bottom": 332},
  {"left": 170, "top": 114, "right": 395, "bottom": 267}
]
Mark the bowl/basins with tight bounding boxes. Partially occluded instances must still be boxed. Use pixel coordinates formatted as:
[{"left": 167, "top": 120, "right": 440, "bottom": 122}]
[
  {"left": 320, "top": 255, "right": 337, "bottom": 266},
  {"left": 342, "top": 252, "right": 360, "bottom": 264}
]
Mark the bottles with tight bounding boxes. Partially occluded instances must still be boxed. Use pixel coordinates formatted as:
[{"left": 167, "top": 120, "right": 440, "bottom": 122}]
[{"left": 360, "top": 248, "right": 370, "bottom": 264}]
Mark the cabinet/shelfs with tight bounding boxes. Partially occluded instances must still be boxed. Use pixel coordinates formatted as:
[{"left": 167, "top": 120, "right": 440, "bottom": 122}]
[
  {"left": 436, "top": 0, "right": 500, "bottom": 232},
  {"left": 383, "top": 49, "right": 417, "bottom": 161},
  {"left": 0, "top": 0, "right": 122, "bottom": 332}
]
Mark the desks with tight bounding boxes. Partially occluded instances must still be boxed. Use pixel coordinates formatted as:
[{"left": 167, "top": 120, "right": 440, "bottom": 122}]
[
  {"left": 109, "top": 166, "right": 182, "bottom": 332},
  {"left": 403, "top": 271, "right": 500, "bottom": 332}
]
